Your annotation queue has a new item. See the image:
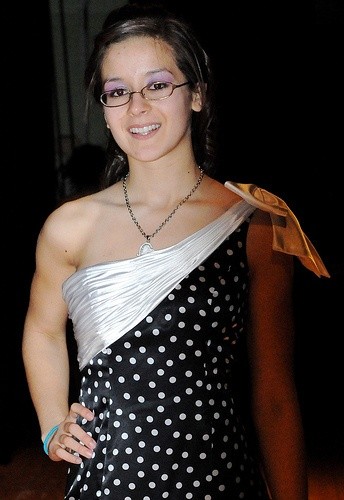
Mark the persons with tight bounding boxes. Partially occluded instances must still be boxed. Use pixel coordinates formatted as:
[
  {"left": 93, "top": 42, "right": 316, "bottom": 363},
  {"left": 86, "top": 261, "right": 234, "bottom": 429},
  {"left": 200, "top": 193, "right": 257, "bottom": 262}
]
[{"left": 22, "top": 7, "right": 331, "bottom": 500}]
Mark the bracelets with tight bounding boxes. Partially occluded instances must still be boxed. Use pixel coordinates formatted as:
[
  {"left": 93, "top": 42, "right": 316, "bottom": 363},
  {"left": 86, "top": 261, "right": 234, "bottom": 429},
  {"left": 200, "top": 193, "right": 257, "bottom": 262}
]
[{"left": 44, "top": 425, "right": 61, "bottom": 456}]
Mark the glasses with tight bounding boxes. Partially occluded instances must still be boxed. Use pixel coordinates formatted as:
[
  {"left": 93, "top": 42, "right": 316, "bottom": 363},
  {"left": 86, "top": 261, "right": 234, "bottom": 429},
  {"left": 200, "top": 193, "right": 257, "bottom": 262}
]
[{"left": 101, "top": 80, "right": 193, "bottom": 108}]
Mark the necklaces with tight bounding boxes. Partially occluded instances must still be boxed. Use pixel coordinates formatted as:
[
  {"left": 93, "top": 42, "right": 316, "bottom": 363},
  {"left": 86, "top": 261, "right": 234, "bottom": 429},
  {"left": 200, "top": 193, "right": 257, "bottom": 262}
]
[{"left": 123, "top": 165, "right": 205, "bottom": 256}]
